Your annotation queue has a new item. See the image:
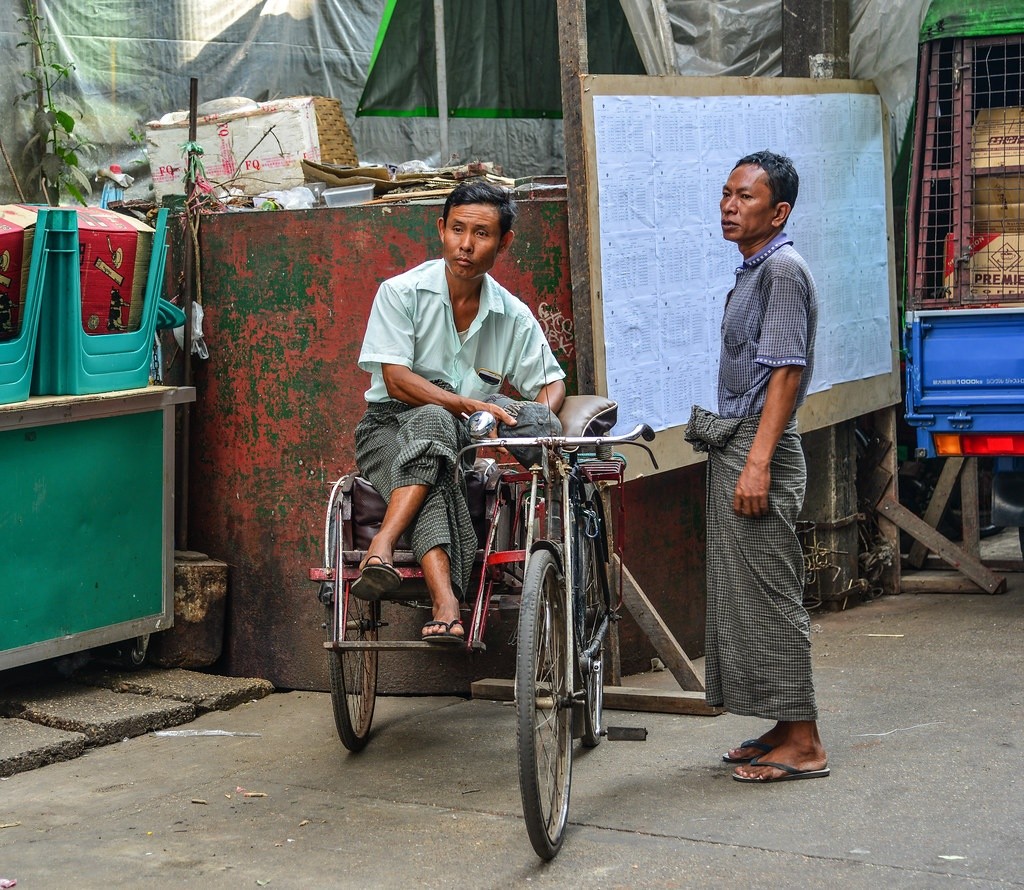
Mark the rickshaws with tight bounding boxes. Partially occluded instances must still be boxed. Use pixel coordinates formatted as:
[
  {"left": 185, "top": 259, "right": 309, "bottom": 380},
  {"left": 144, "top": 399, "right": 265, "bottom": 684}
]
[{"left": 306, "top": 392, "right": 663, "bottom": 863}]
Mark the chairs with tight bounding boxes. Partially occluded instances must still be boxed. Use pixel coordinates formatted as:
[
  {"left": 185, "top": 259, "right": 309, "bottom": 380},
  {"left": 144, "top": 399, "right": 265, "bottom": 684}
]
[{"left": 0, "top": 208, "right": 170, "bottom": 404}]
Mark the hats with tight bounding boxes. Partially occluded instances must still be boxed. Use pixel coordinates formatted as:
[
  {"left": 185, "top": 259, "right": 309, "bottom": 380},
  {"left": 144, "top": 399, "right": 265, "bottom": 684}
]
[{"left": 481, "top": 394, "right": 562, "bottom": 469}]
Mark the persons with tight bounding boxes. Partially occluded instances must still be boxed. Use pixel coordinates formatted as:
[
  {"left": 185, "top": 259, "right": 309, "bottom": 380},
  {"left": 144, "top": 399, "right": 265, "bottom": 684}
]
[
  {"left": 685, "top": 152, "right": 830, "bottom": 782},
  {"left": 350, "top": 182, "right": 566, "bottom": 641}
]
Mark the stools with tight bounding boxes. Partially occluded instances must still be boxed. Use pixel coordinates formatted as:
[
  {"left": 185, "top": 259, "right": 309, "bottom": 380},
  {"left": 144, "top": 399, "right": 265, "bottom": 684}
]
[{"left": 341, "top": 458, "right": 501, "bottom": 550}]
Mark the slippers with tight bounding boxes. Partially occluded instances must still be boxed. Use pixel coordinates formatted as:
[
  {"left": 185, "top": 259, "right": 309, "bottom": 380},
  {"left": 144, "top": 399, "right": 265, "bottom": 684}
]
[
  {"left": 350, "top": 556, "right": 404, "bottom": 602},
  {"left": 421, "top": 619, "right": 464, "bottom": 643},
  {"left": 731, "top": 755, "right": 830, "bottom": 783},
  {"left": 723, "top": 740, "right": 774, "bottom": 763}
]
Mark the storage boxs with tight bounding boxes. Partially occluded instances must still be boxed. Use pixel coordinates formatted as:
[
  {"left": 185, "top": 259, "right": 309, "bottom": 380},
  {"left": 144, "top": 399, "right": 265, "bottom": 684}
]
[
  {"left": 943, "top": 107, "right": 1024, "bottom": 309},
  {"left": 0, "top": 203, "right": 38, "bottom": 341},
  {"left": 20, "top": 204, "right": 156, "bottom": 335}
]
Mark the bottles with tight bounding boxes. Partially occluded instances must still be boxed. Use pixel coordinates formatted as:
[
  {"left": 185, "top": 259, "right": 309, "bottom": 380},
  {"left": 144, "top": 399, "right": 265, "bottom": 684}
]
[{"left": 101, "top": 165, "right": 123, "bottom": 212}]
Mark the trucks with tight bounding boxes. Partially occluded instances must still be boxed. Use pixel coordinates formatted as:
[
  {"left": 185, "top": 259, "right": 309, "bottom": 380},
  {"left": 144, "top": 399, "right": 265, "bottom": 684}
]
[{"left": 895, "top": 308, "right": 1024, "bottom": 567}]
